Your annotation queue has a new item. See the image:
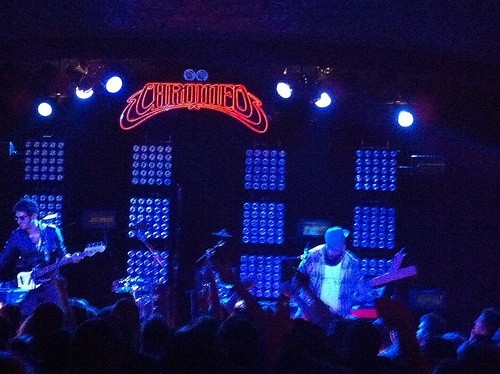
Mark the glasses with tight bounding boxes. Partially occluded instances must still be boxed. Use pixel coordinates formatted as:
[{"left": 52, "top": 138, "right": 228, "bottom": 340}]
[{"left": 14, "top": 215, "right": 28, "bottom": 221}]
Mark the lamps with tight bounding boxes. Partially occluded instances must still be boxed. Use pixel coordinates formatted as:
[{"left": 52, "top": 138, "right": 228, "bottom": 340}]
[{"left": 275, "top": 64, "right": 309, "bottom": 100}]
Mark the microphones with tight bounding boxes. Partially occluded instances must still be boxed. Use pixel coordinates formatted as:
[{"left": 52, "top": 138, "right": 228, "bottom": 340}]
[
  {"left": 43, "top": 213, "right": 58, "bottom": 221},
  {"left": 217, "top": 240, "right": 227, "bottom": 246},
  {"left": 303, "top": 243, "right": 309, "bottom": 259}
]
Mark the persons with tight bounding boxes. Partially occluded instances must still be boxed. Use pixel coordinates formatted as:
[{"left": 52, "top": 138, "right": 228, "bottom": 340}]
[
  {"left": 0, "top": 195, "right": 83, "bottom": 335},
  {"left": 0, "top": 290, "right": 499, "bottom": 373},
  {"left": 288, "top": 225, "right": 362, "bottom": 332}
]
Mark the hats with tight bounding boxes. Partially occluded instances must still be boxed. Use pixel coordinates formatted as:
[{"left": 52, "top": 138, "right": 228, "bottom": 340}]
[{"left": 324, "top": 226, "right": 350, "bottom": 246}]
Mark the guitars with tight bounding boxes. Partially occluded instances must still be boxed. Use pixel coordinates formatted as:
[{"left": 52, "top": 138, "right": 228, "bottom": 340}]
[{"left": 0, "top": 241, "right": 107, "bottom": 304}]
